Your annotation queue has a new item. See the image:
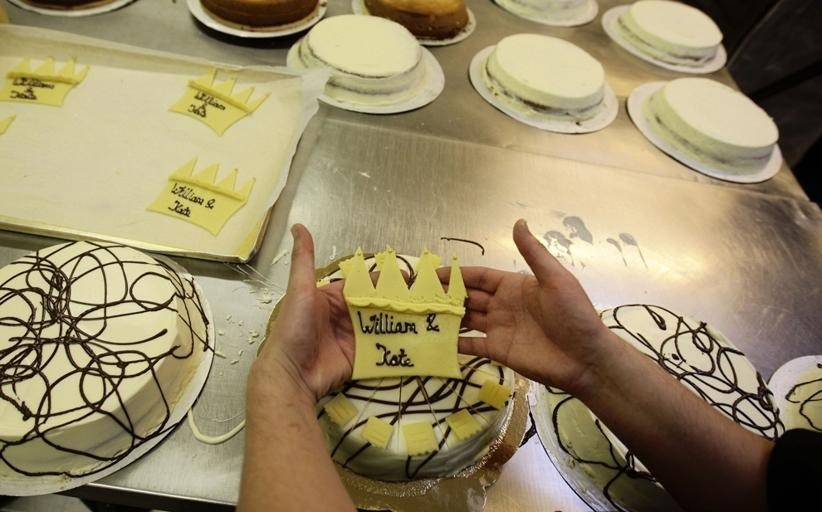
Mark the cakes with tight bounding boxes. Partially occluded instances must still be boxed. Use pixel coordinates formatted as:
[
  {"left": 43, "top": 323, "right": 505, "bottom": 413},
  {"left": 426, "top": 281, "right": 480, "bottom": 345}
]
[
  {"left": 316, "top": 255, "right": 514, "bottom": 484},
  {"left": 20, "top": 0, "right": 114, "bottom": 10},
  {"left": 0, "top": 239, "right": 209, "bottom": 482},
  {"left": 500, "top": 0, "right": 590, "bottom": 21},
  {"left": 645, "top": 78, "right": 779, "bottom": 175},
  {"left": 299, "top": 14, "right": 427, "bottom": 107},
  {"left": 785, "top": 368, "right": 821, "bottom": 431},
  {"left": 537, "top": 303, "right": 785, "bottom": 512},
  {"left": 613, "top": 0, "right": 723, "bottom": 69},
  {"left": 364, "top": 0, "right": 468, "bottom": 40},
  {"left": 200, "top": 0, "right": 320, "bottom": 31},
  {"left": 480, "top": 34, "right": 605, "bottom": 125}
]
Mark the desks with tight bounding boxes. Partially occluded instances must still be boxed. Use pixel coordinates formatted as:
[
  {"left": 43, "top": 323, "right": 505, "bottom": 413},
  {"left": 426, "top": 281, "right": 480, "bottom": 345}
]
[{"left": 3, "top": 0, "right": 820, "bottom": 511}]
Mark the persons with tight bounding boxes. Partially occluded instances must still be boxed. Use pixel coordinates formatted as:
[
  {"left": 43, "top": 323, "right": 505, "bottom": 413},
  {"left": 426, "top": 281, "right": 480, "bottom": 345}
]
[{"left": 235, "top": 218, "right": 822, "bottom": 512}]
